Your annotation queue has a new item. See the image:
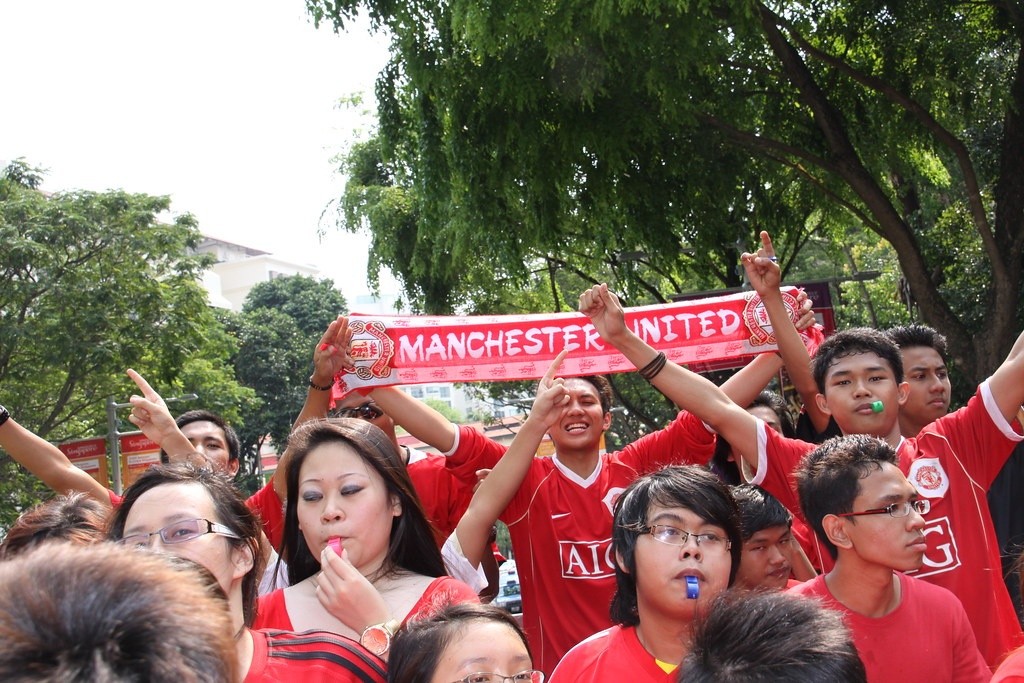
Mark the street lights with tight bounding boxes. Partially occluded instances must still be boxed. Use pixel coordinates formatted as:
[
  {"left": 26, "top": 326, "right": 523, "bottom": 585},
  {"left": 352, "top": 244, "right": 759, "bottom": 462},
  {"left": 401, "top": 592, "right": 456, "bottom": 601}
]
[{"left": 105, "top": 389, "right": 198, "bottom": 498}]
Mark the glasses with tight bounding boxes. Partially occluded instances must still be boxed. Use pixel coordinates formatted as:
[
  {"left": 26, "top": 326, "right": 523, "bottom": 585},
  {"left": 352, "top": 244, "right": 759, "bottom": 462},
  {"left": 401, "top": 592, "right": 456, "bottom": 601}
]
[
  {"left": 112, "top": 518, "right": 242, "bottom": 550},
  {"left": 452, "top": 668, "right": 545, "bottom": 683},
  {"left": 636, "top": 526, "right": 733, "bottom": 556},
  {"left": 837, "top": 499, "right": 932, "bottom": 518},
  {"left": 332, "top": 400, "right": 383, "bottom": 422}
]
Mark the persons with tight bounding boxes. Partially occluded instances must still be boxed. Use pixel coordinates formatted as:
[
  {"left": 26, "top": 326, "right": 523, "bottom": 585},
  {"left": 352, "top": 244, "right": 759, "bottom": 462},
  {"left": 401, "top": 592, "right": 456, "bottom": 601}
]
[{"left": 0, "top": 230, "right": 1024, "bottom": 683}]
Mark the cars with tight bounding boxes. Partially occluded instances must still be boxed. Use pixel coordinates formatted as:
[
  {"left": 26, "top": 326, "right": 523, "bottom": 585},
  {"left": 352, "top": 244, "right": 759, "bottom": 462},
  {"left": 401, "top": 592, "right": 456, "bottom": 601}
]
[{"left": 490, "top": 559, "right": 523, "bottom": 616}]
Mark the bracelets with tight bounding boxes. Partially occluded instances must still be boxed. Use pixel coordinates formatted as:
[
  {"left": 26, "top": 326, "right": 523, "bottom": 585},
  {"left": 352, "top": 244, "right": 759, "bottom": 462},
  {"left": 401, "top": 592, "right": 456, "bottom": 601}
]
[
  {"left": 309, "top": 375, "right": 334, "bottom": 391},
  {"left": 0, "top": 405, "right": 12, "bottom": 430},
  {"left": 639, "top": 351, "right": 669, "bottom": 380}
]
